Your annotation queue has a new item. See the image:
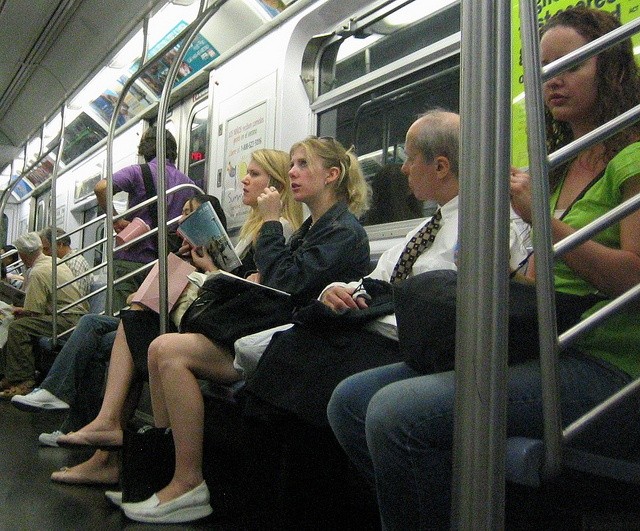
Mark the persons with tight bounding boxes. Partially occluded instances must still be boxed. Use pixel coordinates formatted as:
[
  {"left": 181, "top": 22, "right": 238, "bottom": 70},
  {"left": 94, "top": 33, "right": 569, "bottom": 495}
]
[
  {"left": 51, "top": 148, "right": 304, "bottom": 485},
  {"left": 42, "top": 226, "right": 91, "bottom": 300},
  {"left": 0, "top": 232, "right": 88, "bottom": 395},
  {"left": 242, "top": 112, "right": 536, "bottom": 497},
  {"left": 106, "top": 139, "right": 370, "bottom": 522},
  {"left": 97, "top": 127, "right": 196, "bottom": 314},
  {"left": 0, "top": 245, "right": 17, "bottom": 265},
  {"left": 327, "top": 7, "right": 639, "bottom": 528},
  {"left": 12, "top": 195, "right": 229, "bottom": 446}
]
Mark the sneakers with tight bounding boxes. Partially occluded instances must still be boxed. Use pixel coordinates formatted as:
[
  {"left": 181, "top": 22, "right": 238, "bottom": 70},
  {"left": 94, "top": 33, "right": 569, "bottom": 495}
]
[
  {"left": 12, "top": 388, "right": 71, "bottom": 409},
  {"left": 122, "top": 479, "right": 212, "bottom": 523},
  {"left": 0, "top": 380, "right": 37, "bottom": 401},
  {"left": 104, "top": 490, "right": 122, "bottom": 507},
  {"left": 56, "top": 429, "right": 123, "bottom": 451},
  {"left": 50, "top": 466, "right": 122, "bottom": 484},
  {"left": 40, "top": 431, "right": 75, "bottom": 446}
]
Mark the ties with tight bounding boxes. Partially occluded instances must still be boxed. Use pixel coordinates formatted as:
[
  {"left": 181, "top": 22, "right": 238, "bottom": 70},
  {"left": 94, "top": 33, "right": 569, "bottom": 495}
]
[{"left": 391, "top": 208, "right": 444, "bottom": 285}]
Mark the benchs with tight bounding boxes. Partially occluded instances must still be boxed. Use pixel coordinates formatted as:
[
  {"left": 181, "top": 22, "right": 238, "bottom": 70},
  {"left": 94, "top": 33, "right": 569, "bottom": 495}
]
[
  {"left": 199, "top": 248, "right": 640, "bottom": 488},
  {"left": 31, "top": 274, "right": 109, "bottom": 358}
]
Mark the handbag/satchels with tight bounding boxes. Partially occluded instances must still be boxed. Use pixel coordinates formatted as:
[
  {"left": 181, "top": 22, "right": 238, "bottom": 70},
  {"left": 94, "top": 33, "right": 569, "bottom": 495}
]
[
  {"left": 394, "top": 169, "right": 612, "bottom": 375},
  {"left": 245, "top": 319, "right": 380, "bottom": 427},
  {"left": 181, "top": 276, "right": 291, "bottom": 349}
]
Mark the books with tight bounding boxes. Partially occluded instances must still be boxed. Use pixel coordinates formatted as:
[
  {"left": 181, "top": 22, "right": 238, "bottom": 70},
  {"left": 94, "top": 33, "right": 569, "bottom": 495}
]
[
  {"left": 186, "top": 270, "right": 291, "bottom": 296},
  {"left": 175, "top": 201, "right": 242, "bottom": 273}
]
